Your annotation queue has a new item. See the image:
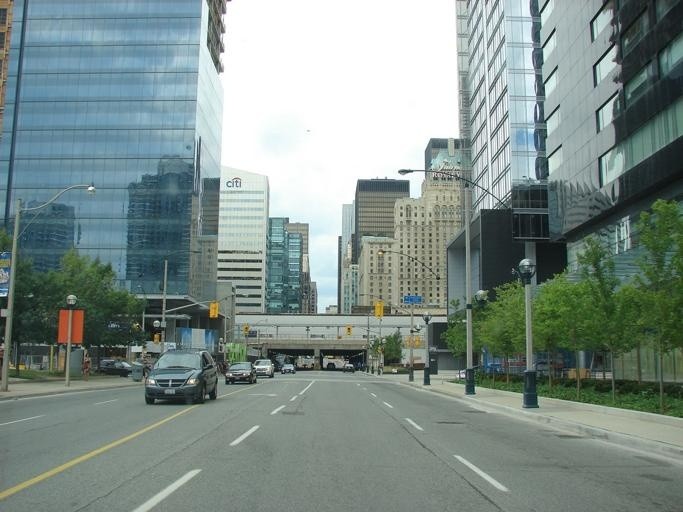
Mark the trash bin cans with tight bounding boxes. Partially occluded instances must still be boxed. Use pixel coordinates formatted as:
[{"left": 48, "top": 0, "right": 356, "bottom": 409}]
[
  {"left": 132, "top": 364, "right": 144, "bottom": 383},
  {"left": 562, "top": 368, "right": 577, "bottom": 378}
]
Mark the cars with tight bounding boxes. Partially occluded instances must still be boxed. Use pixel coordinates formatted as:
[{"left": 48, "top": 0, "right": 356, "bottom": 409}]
[
  {"left": 343, "top": 364, "right": 355, "bottom": 373},
  {"left": 327, "top": 362, "right": 335, "bottom": 370},
  {"left": 144, "top": 349, "right": 218, "bottom": 404},
  {"left": 225, "top": 362, "right": 257, "bottom": 384},
  {"left": 95, "top": 358, "right": 160, "bottom": 378},
  {"left": 281, "top": 364, "right": 295, "bottom": 375}
]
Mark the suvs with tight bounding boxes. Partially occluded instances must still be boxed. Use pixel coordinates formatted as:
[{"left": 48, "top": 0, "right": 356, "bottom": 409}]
[{"left": 252, "top": 359, "right": 275, "bottom": 378}]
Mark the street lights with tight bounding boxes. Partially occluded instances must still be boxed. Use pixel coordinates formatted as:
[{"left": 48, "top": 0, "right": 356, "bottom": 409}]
[
  {"left": 516, "top": 259, "right": 539, "bottom": 408},
  {"left": 65, "top": 294, "right": 77, "bottom": 387},
  {"left": 423, "top": 311, "right": 433, "bottom": 385},
  {"left": 161, "top": 292, "right": 274, "bottom": 362},
  {"left": 475, "top": 289, "right": 487, "bottom": 311},
  {"left": 351, "top": 294, "right": 421, "bottom": 381},
  {"left": 1, "top": 183, "right": 96, "bottom": 391}
]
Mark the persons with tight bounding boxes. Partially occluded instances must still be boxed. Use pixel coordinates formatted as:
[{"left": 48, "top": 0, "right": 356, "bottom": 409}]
[{"left": 82, "top": 358, "right": 90, "bottom": 377}]
